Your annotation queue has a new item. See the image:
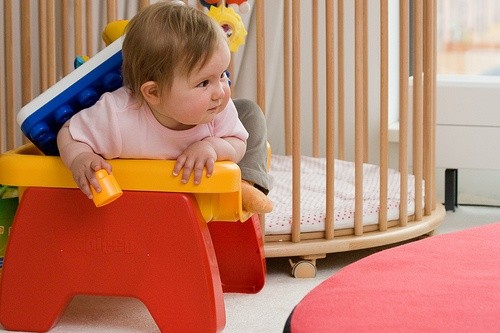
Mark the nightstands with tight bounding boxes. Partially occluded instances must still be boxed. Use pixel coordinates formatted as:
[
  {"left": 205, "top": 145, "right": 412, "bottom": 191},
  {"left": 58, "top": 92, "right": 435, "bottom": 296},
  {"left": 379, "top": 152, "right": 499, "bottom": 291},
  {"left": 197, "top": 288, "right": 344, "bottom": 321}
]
[{"left": 407, "top": 74, "right": 499, "bottom": 208}]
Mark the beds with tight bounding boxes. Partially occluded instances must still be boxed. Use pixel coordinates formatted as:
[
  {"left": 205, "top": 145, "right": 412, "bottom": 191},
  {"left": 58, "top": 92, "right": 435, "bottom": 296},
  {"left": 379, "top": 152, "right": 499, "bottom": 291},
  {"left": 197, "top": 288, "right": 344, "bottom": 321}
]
[{"left": 0, "top": 0, "right": 447, "bottom": 278}]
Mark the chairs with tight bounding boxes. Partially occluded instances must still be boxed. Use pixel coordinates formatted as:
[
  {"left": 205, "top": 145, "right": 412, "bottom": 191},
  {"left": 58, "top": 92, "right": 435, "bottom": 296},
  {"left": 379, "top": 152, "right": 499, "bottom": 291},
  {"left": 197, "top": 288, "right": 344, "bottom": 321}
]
[{"left": 0, "top": 143, "right": 271, "bottom": 333}]
